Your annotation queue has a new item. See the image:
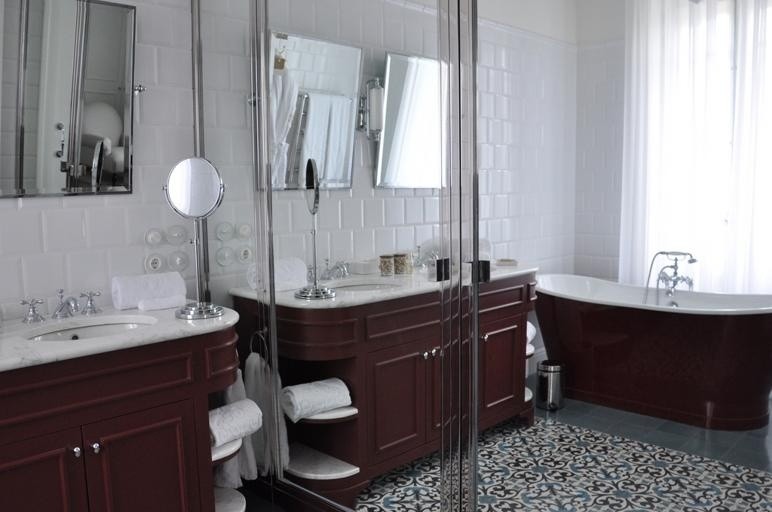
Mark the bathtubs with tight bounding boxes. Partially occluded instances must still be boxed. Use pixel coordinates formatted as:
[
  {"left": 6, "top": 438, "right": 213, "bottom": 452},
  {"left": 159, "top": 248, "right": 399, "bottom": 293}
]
[{"left": 534, "top": 273, "right": 772, "bottom": 430}]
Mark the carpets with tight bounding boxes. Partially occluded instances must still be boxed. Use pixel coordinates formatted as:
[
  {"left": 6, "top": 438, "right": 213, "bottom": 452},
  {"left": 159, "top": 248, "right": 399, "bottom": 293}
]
[{"left": 352, "top": 415, "right": 771, "bottom": 512}]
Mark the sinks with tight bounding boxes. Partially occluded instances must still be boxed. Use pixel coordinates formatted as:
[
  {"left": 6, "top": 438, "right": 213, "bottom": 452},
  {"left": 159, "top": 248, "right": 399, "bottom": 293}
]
[
  {"left": 328, "top": 278, "right": 404, "bottom": 296},
  {"left": 19, "top": 314, "right": 159, "bottom": 343}
]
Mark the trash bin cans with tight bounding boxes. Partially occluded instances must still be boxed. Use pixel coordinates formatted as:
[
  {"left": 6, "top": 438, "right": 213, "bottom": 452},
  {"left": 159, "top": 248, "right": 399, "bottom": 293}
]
[{"left": 535, "top": 360, "right": 566, "bottom": 411}]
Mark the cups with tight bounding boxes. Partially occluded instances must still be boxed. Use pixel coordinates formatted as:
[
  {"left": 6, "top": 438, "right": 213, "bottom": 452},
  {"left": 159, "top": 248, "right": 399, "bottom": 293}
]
[
  {"left": 394, "top": 254, "right": 408, "bottom": 275},
  {"left": 380, "top": 255, "right": 396, "bottom": 277}
]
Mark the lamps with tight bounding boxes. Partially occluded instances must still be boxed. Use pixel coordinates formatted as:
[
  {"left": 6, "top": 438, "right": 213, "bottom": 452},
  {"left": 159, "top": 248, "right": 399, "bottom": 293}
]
[{"left": 359, "top": 77, "right": 386, "bottom": 142}]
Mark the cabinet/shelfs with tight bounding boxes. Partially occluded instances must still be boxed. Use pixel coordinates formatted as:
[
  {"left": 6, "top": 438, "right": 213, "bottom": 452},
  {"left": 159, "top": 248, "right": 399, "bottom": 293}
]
[
  {"left": 524, "top": 344, "right": 536, "bottom": 402},
  {"left": 282, "top": 406, "right": 360, "bottom": 493},
  {"left": 210, "top": 436, "right": 248, "bottom": 512},
  {"left": 0, "top": 332, "right": 210, "bottom": 512},
  {"left": 358, "top": 273, "right": 529, "bottom": 483}
]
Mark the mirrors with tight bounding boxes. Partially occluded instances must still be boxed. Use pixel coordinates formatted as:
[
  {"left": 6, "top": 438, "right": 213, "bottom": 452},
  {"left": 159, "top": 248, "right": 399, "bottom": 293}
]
[
  {"left": 264, "top": 31, "right": 364, "bottom": 188},
  {"left": 1, "top": 0, "right": 135, "bottom": 197},
  {"left": 188, "top": 0, "right": 772, "bottom": 512},
  {"left": 372, "top": 51, "right": 452, "bottom": 190},
  {"left": 92, "top": 138, "right": 105, "bottom": 194},
  {"left": 164, "top": 156, "right": 226, "bottom": 319},
  {"left": 294, "top": 160, "right": 336, "bottom": 300}
]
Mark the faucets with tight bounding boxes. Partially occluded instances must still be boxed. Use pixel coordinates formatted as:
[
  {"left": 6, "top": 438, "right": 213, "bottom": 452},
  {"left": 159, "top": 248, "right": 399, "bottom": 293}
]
[
  {"left": 323, "top": 259, "right": 349, "bottom": 281},
  {"left": 52, "top": 289, "right": 80, "bottom": 317}
]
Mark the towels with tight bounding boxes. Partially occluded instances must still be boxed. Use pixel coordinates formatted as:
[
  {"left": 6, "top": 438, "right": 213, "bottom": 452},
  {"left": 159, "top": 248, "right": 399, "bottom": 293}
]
[
  {"left": 111, "top": 272, "right": 188, "bottom": 311},
  {"left": 247, "top": 253, "right": 308, "bottom": 295},
  {"left": 525, "top": 320, "right": 537, "bottom": 356},
  {"left": 277, "top": 372, "right": 353, "bottom": 480},
  {"left": 208, "top": 352, "right": 271, "bottom": 488},
  {"left": 299, "top": 90, "right": 355, "bottom": 185}
]
